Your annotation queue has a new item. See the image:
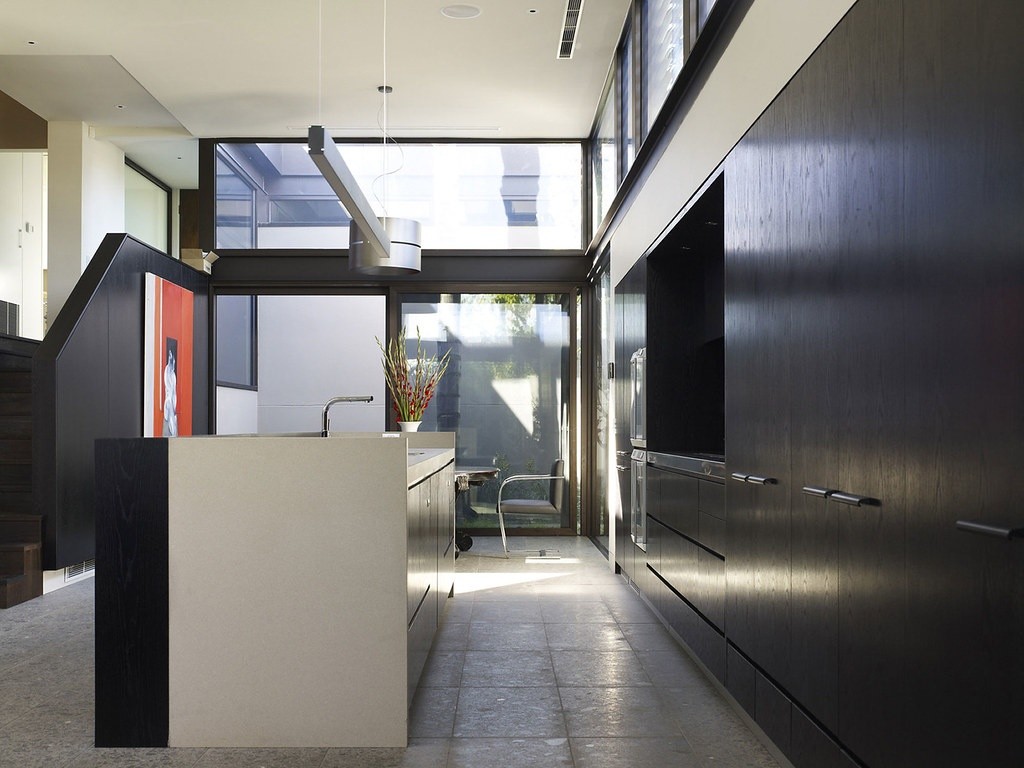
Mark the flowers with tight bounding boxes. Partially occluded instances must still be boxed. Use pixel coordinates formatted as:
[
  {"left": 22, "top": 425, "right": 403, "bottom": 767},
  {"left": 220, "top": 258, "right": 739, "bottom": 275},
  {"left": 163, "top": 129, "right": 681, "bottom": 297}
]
[{"left": 374, "top": 320, "right": 453, "bottom": 421}]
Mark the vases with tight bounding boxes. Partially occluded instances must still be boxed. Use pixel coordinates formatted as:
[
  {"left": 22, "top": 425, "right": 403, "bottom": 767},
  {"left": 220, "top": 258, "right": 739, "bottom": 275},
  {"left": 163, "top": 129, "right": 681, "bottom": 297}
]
[{"left": 397, "top": 421, "right": 423, "bottom": 433}]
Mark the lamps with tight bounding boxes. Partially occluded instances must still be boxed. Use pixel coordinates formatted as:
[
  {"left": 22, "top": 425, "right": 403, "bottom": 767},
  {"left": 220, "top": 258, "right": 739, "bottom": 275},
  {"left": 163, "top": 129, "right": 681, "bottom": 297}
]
[
  {"left": 307, "top": 0, "right": 390, "bottom": 258},
  {"left": 348, "top": 84, "right": 422, "bottom": 275}
]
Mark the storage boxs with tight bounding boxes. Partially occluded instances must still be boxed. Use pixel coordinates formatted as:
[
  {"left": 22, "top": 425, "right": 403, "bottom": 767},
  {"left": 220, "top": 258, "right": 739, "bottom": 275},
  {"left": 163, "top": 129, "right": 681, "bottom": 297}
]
[{"left": 181, "top": 249, "right": 220, "bottom": 275}]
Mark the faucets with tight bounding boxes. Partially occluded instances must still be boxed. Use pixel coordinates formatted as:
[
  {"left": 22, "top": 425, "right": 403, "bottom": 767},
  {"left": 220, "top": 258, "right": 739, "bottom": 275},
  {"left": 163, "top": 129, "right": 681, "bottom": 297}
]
[{"left": 321, "top": 395, "right": 374, "bottom": 437}]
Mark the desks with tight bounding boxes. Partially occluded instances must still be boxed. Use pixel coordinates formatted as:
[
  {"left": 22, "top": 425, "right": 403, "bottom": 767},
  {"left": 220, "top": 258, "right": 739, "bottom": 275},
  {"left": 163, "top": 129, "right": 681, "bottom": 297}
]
[{"left": 455, "top": 466, "right": 500, "bottom": 556}]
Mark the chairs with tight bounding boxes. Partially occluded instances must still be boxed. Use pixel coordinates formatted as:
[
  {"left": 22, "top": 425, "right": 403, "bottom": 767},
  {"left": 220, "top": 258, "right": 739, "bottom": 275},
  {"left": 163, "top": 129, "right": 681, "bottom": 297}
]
[{"left": 497, "top": 457, "right": 565, "bottom": 560}]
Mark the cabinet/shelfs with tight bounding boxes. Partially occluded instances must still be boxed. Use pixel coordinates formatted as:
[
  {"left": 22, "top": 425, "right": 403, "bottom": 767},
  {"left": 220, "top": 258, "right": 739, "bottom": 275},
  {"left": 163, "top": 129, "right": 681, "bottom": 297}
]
[
  {"left": 724, "top": 0, "right": 1024, "bottom": 768},
  {"left": 95, "top": 467, "right": 455, "bottom": 747},
  {"left": 615, "top": 251, "right": 646, "bottom": 588}
]
